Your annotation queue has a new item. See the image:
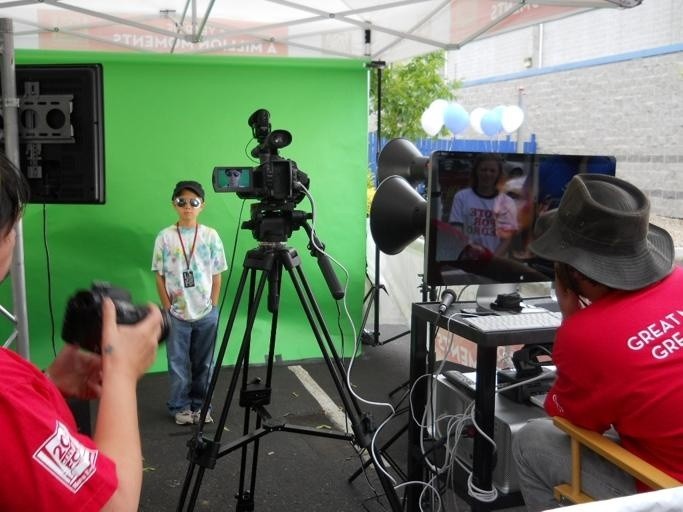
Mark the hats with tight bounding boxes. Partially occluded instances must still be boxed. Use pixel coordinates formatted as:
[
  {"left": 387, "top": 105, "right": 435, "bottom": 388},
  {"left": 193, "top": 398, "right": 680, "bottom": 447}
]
[
  {"left": 526, "top": 174, "right": 675, "bottom": 291},
  {"left": 172, "top": 181, "right": 204, "bottom": 202}
]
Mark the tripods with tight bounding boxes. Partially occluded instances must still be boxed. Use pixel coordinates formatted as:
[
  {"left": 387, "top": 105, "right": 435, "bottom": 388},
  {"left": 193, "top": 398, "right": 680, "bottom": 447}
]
[{"left": 175, "top": 243, "right": 402, "bottom": 511}]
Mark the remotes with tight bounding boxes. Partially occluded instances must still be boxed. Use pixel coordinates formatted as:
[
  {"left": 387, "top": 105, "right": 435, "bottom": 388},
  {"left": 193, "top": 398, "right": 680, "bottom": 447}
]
[{"left": 445, "top": 369, "right": 476, "bottom": 394}]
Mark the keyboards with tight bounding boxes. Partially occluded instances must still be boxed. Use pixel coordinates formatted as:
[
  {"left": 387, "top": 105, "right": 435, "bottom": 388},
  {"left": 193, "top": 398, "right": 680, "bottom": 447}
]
[{"left": 462, "top": 311, "right": 564, "bottom": 333}]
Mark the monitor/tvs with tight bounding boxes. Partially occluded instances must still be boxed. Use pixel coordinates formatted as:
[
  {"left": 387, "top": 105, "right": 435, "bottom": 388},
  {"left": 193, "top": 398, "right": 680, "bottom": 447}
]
[
  {"left": 0, "top": 63, "right": 105, "bottom": 207},
  {"left": 424, "top": 150, "right": 616, "bottom": 316}
]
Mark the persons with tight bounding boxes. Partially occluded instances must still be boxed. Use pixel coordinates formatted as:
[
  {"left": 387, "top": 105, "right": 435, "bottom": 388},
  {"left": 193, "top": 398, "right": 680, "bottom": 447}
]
[
  {"left": 149, "top": 179, "right": 229, "bottom": 428},
  {"left": 492, "top": 162, "right": 553, "bottom": 259},
  {"left": 1, "top": 151, "right": 167, "bottom": 512},
  {"left": 222, "top": 169, "right": 244, "bottom": 188},
  {"left": 509, "top": 171, "right": 683, "bottom": 511},
  {"left": 448, "top": 157, "right": 519, "bottom": 259}
]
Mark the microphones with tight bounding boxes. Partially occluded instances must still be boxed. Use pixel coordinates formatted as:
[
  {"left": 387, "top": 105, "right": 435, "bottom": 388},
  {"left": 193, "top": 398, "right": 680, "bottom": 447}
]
[{"left": 439, "top": 289, "right": 458, "bottom": 314}]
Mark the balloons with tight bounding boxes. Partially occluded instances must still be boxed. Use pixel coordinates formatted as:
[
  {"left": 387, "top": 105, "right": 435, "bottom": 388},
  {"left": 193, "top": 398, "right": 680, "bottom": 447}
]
[{"left": 421, "top": 98, "right": 525, "bottom": 137}]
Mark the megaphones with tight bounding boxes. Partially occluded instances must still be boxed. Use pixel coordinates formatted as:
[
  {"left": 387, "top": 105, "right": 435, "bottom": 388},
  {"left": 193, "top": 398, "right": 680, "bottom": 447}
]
[
  {"left": 374, "top": 139, "right": 429, "bottom": 189},
  {"left": 370, "top": 175, "right": 427, "bottom": 255}
]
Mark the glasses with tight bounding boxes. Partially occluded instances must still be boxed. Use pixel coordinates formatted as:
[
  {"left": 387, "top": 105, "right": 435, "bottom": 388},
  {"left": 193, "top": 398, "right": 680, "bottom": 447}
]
[{"left": 174, "top": 197, "right": 201, "bottom": 207}]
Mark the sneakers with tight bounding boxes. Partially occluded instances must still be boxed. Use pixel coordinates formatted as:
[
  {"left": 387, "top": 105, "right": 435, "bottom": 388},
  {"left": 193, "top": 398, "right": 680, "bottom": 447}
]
[
  {"left": 175, "top": 410, "right": 194, "bottom": 424},
  {"left": 192, "top": 408, "right": 214, "bottom": 424}
]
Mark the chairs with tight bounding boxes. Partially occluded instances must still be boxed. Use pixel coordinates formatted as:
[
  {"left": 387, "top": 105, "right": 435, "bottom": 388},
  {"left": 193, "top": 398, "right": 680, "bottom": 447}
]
[{"left": 552, "top": 414, "right": 683, "bottom": 506}]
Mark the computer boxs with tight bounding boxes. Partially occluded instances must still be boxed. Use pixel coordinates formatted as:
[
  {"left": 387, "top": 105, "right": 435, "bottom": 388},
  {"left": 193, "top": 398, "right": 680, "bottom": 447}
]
[{"left": 426, "top": 372, "right": 551, "bottom": 496}]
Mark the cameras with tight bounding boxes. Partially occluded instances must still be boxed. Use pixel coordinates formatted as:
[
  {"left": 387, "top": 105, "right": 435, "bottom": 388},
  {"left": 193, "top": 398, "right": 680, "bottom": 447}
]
[{"left": 59, "top": 280, "right": 171, "bottom": 357}]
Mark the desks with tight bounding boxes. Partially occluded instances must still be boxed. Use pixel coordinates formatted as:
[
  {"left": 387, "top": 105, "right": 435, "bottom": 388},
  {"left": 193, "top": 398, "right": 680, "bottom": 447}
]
[{"left": 407, "top": 293, "right": 566, "bottom": 512}]
